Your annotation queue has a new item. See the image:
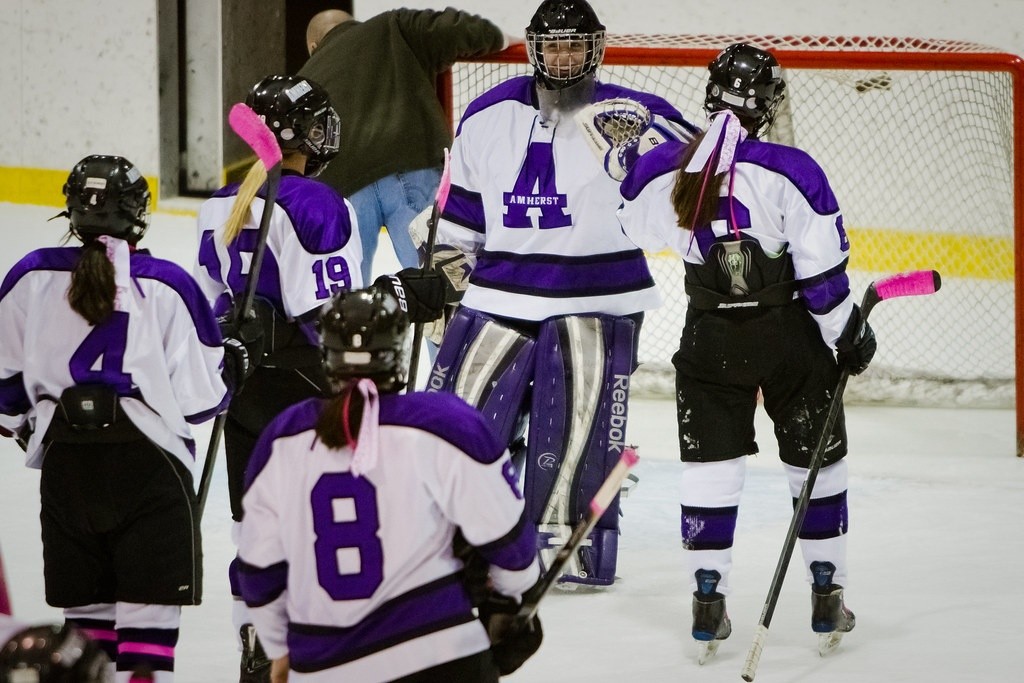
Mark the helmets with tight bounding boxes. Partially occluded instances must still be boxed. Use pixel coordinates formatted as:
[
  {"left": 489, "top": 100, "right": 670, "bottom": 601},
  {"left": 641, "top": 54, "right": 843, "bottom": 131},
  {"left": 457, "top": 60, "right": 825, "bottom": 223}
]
[
  {"left": 525, "top": 0, "right": 606, "bottom": 90},
  {"left": 0, "top": 626, "right": 112, "bottom": 683},
  {"left": 316, "top": 286, "right": 410, "bottom": 394},
  {"left": 702, "top": 43, "right": 786, "bottom": 137},
  {"left": 62, "top": 154, "right": 152, "bottom": 245},
  {"left": 245, "top": 74, "right": 342, "bottom": 177}
]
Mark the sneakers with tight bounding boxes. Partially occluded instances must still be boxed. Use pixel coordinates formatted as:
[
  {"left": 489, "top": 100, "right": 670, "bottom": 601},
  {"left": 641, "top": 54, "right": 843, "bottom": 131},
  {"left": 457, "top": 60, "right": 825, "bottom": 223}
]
[
  {"left": 809, "top": 560, "right": 856, "bottom": 657},
  {"left": 238, "top": 623, "right": 271, "bottom": 683},
  {"left": 691, "top": 568, "right": 732, "bottom": 665}
]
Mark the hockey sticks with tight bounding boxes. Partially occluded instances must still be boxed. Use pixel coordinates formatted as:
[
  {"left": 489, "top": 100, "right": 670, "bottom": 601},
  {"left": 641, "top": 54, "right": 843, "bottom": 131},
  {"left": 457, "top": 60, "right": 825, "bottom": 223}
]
[
  {"left": 406, "top": 145, "right": 454, "bottom": 398},
  {"left": 198, "top": 102, "right": 284, "bottom": 523},
  {"left": 741, "top": 269, "right": 943, "bottom": 680},
  {"left": 509, "top": 447, "right": 642, "bottom": 636}
]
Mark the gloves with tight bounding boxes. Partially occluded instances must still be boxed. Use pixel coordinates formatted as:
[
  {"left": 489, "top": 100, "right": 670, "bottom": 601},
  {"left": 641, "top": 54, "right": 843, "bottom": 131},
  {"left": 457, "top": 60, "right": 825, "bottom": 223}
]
[
  {"left": 373, "top": 266, "right": 450, "bottom": 325},
  {"left": 806, "top": 287, "right": 877, "bottom": 376},
  {"left": 422, "top": 244, "right": 472, "bottom": 347},
  {"left": 219, "top": 288, "right": 267, "bottom": 384}
]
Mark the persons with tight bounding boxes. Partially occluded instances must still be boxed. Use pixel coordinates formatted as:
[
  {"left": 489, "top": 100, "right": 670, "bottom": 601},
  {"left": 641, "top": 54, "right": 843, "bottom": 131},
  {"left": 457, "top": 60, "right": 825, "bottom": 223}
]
[
  {"left": 293, "top": 9, "right": 528, "bottom": 369},
  {"left": 615, "top": 0, "right": 878, "bottom": 668},
  {"left": 406, "top": 0, "right": 706, "bottom": 594},
  {"left": 0, "top": 72, "right": 548, "bottom": 683}
]
